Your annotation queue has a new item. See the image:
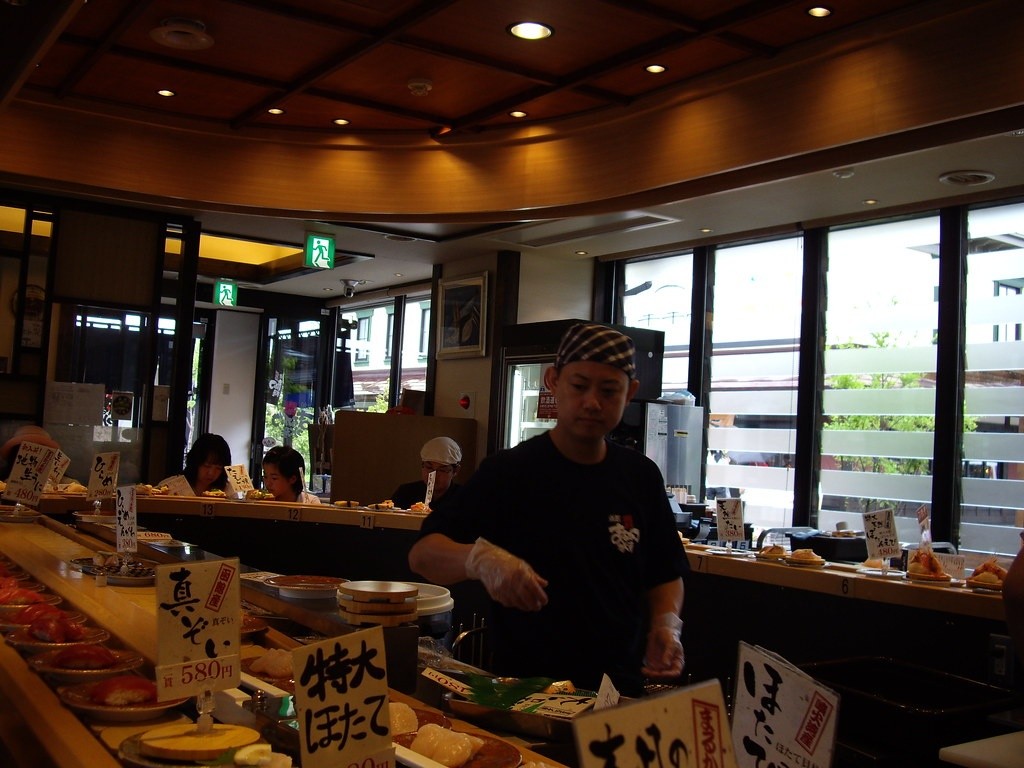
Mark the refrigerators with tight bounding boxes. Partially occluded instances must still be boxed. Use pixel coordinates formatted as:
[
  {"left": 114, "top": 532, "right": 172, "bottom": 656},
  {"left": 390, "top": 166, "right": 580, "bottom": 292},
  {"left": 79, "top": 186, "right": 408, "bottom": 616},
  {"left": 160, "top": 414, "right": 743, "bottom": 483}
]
[{"left": 495, "top": 318, "right": 665, "bottom": 487}]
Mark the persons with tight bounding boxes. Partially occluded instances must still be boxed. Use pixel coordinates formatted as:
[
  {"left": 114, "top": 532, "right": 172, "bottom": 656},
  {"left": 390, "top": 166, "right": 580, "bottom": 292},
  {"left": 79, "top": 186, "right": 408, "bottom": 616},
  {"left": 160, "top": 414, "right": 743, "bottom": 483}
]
[
  {"left": 388, "top": 435, "right": 464, "bottom": 512},
  {"left": 1000, "top": 528, "right": 1024, "bottom": 733},
  {"left": 261, "top": 444, "right": 322, "bottom": 506},
  {"left": 405, "top": 321, "right": 698, "bottom": 700},
  {"left": 156, "top": 432, "right": 237, "bottom": 499},
  {"left": 0, "top": 424, "right": 82, "bottom": 489}
]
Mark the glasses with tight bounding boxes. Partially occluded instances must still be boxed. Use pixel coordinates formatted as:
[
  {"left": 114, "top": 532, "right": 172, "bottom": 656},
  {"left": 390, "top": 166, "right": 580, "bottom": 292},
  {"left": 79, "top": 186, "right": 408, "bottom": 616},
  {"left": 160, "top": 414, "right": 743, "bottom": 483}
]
[{"left": 422, "top": 461, "right": 455, "bottom": 474}]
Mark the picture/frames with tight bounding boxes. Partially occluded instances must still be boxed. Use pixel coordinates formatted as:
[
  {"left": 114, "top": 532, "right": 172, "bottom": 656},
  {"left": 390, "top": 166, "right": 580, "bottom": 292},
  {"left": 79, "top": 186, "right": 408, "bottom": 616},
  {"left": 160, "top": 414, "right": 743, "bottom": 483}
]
[{"left": 433, "top": 270, "right": 486, "bottom": 358}]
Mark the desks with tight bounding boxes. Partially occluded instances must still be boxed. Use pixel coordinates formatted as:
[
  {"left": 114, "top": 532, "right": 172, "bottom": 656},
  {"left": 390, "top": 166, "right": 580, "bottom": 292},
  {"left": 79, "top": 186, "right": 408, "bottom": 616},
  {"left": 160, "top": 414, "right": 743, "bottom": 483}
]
[{"left": 61, "top": 493, "right": 1013, "bottom": 624}]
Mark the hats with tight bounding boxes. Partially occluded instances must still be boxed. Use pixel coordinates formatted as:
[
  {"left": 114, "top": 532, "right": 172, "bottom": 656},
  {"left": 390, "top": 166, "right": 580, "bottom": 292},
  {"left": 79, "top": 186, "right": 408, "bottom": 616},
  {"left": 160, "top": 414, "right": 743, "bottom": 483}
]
[
  {"left": 557, "top": 323, "right": 636, "bottom": 380},
  {"left": 420, "top": 437, "right": 462, "bottom": 465}
]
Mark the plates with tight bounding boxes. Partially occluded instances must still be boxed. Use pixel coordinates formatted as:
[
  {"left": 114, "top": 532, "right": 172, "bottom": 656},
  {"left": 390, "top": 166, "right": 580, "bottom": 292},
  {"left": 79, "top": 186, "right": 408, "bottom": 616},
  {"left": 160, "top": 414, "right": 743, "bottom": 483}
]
[
  {"left": 707, "top": 547, "right": 1001, "bottom": 592},
  {"left": 240, "top": 656, "right": 522, "bottom": 767},
  {"left": 0, "top": 505, "right": 42, "bottom": 523},
  {"left": 0, "top": 558, "right": 269, "bottom": 768},
  {"left": 264, "top": 575, "right": 351, "bottom": 599},
  {"left": 365, "top": 506, "right": 401, "bottom": 512}
]
